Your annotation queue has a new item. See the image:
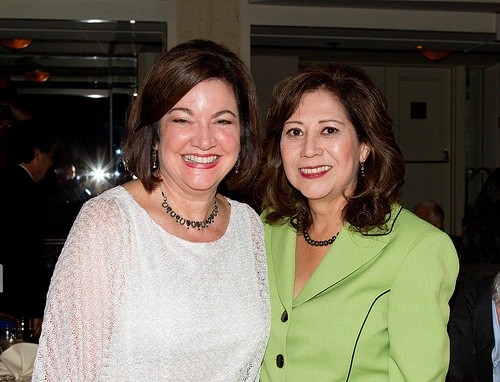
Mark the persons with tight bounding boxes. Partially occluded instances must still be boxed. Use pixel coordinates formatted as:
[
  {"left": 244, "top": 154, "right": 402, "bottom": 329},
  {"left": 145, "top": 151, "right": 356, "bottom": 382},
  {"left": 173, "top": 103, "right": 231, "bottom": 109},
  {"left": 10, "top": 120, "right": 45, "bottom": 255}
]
[
  {"left": 32, "top": 40, "right": 270, "bottom": 382},
  {"left": 0, "top": 107, "right": 132, "bottom": 343},
  {"left": 414, "top": 199, "right": 500, "bottom": 382},
  {"left": 258, "top": 64, "right": 459, "bottom": 382}
]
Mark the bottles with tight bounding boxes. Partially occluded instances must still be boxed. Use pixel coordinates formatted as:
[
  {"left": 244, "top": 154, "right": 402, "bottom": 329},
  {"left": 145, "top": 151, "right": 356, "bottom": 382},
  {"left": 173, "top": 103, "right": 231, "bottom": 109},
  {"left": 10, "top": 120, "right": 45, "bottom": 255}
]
[{"left": 5, "top": 324, "right": 20, "bottom": 343}]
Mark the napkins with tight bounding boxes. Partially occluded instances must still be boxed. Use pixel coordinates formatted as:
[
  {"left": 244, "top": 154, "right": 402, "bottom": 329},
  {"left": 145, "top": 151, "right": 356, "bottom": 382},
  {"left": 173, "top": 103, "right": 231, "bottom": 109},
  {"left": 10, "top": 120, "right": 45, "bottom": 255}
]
[{"left": 0, "top": 342, "right": 39, "bottom": 382}]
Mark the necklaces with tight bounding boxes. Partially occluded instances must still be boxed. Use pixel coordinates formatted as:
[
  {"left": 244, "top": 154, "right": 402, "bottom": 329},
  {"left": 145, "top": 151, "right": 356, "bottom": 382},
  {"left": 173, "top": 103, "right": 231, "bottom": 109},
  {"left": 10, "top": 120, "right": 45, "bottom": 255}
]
[
  {"left": 159, "top": 184, "right": 218, "bottom": 230},
  {"left": 303, "top": 226, "right": 339, "bottom": 246}
]
